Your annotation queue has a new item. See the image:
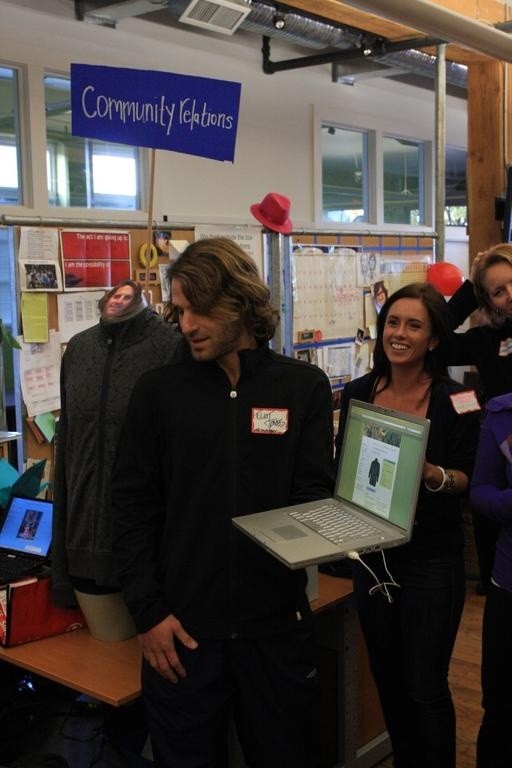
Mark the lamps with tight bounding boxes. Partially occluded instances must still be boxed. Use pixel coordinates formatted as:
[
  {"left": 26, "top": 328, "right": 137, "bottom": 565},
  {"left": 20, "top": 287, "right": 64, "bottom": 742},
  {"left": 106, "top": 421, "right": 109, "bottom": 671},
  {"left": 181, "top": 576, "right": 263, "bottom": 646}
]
[
  {"left": 360, "top": 39, "right": 372, "bottom": 56},
  {"left": 273, "top": 15, "right": 286, "bottom": 31}
]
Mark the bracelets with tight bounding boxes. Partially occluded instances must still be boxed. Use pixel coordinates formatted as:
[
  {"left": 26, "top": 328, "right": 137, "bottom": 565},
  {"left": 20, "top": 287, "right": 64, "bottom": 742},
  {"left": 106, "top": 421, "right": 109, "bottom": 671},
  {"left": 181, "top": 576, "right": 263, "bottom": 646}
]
[{"left": 427, "top": 463, "right": 455, "bottom": 498}]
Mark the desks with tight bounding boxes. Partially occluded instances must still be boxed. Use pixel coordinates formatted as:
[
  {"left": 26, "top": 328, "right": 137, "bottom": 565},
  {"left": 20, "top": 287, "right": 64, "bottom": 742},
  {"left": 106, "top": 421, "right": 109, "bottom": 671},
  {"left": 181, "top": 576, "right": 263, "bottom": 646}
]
[{"left": 0, "top": 569, "right": 353, "bottom": 768}]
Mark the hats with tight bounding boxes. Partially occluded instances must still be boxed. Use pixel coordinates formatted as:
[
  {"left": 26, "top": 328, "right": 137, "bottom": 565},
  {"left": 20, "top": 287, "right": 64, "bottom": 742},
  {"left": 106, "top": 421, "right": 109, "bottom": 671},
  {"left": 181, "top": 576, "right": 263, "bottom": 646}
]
[{"left": 250, "top": 192, "right": 293, "bottom": 234}]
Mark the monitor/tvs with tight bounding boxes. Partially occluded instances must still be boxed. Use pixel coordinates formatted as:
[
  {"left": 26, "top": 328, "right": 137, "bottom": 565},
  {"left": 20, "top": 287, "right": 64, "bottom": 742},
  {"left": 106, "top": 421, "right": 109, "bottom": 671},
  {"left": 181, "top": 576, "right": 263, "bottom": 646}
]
[
  {"left": 0, "top": 494, "right": 54, "bottom": 586},
  {"left": 232, "top": 399, "right": 432, "bottom": 570}
]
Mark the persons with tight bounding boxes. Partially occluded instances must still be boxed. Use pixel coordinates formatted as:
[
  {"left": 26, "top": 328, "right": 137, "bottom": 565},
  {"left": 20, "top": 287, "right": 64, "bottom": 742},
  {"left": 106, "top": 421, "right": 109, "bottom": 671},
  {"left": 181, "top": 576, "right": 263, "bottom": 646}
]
[
  {"left": 98, "top": 279, "right": 139, "bottom": 315},
  {"left": 445, "top": 243, "right": 512, "bottom": 595},
  {"left": 108, "top": 234, "right": 333, "bottom": 766},
  {"left": 333, "top": 280, "right": 479, "bottom": 766},
  {"left": 51, "top": 300, "right": 184, "bottom": 605},
  {"left": 466, "top": 391, "right": 512, "bottom": 767},
  {"left": 26, "top": 266, "right": 56, "bottom": 288}
]
